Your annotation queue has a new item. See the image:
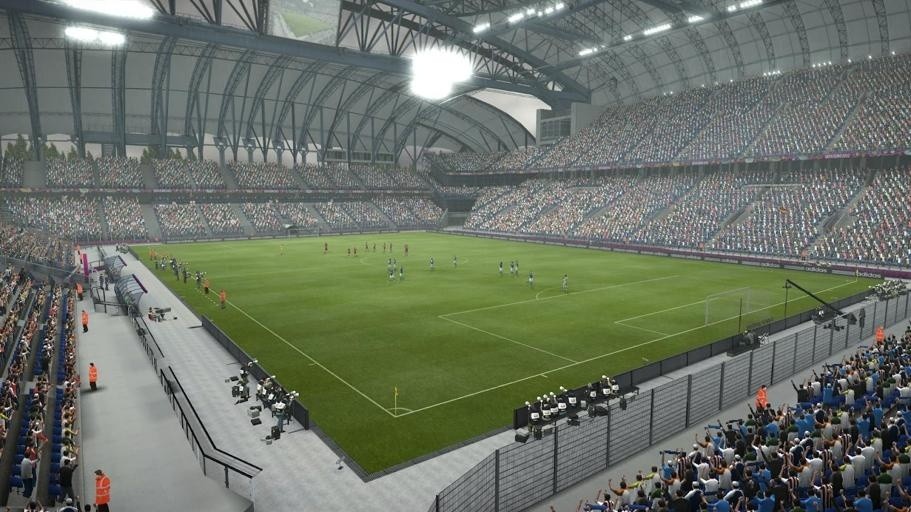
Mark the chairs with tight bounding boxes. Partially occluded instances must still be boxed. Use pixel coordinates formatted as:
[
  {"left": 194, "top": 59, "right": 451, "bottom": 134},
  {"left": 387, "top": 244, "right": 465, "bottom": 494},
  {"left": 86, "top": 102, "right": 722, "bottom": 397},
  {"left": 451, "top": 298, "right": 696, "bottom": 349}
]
[{"left": 1, "top": 205, "right": 83, "bottom": 510}]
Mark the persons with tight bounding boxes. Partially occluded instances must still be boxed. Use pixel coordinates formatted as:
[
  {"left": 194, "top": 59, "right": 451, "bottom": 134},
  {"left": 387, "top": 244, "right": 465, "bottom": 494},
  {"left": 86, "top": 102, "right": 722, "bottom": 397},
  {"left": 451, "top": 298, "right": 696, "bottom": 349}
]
[
  {"left": 149, "top": 306, "right": 161, "bottom": 321},
  {"left": 387, "top": 258, "right": 404, "bottom": 282},
  {"left": 561, "top": 273, "right": 568, "bottom": 289},
  {"left": 348, "top": 247, "right": 351, "bottom": 256},
  {"left": 354, "top": 247, "right": 357, "bottom": 256},
  {"left": 324, "top": 241, "right": 328, "bottom": 253},
  {"left": 529, "top": 271, "right": 535, "bottom": 288},
  {"left": 232, "top": 360, "right": 296, "bottom": 439},
  {"left": 453, "top": 256, "right": 457, "bottom": 268},
  {"left": 0, "top": 239, "right": 131, "bottom": 512},
  {"left": 405, "top": 243, "right": 409, "bottom": 256},
  {"left": 430, "top": 256, "right": 434, "bottom": 269},
  {"left": 148, "top": 247, "right": 227, "bottom": 309}
]
